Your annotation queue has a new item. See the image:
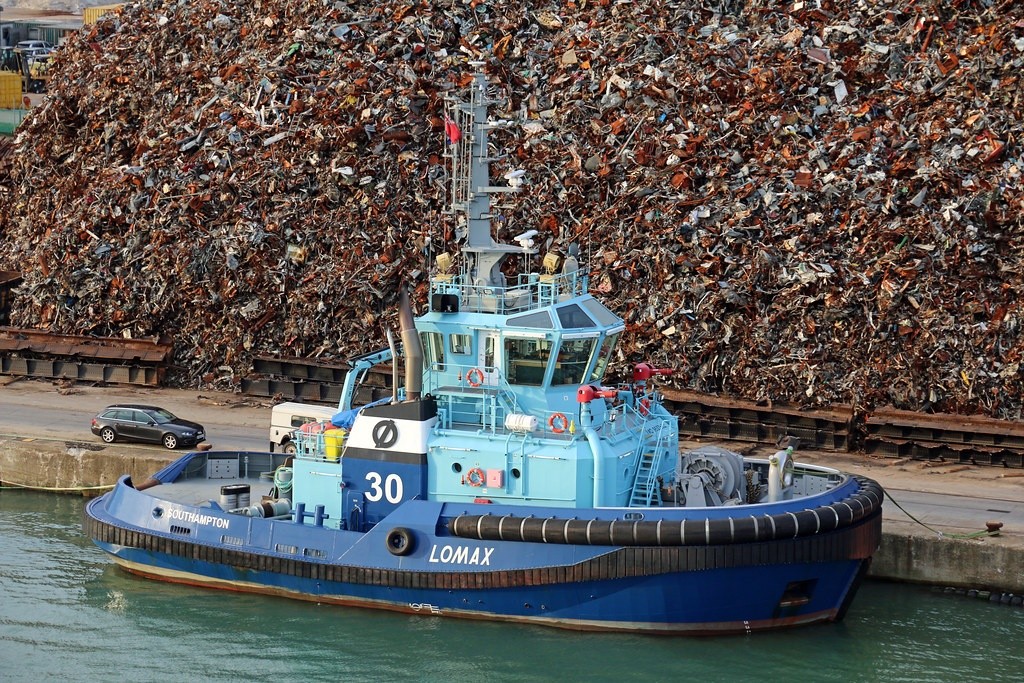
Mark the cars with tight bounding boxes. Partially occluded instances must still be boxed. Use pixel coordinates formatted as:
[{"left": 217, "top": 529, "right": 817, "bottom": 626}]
[{"left": 14, "top": 38, "right": 61, "bottom": 94}]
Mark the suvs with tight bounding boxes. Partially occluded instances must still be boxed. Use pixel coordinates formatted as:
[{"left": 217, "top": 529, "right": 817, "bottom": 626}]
[{"left": 90, "top": 403, "right": 206, "bottom": 449}]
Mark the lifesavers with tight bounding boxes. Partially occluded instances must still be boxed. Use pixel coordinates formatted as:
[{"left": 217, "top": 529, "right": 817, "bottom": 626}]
[
  {"left": 466, "top": 369, "right": 484, "bottom": 386},
  {"left": 549, "top": 413, "right": 567, "bottom": 433},
  {"left": 386, "top": 527, "right": 412, "bottom": 555},
  {"left": 467, "top": 467, "right": 485, "bottom": 487}
]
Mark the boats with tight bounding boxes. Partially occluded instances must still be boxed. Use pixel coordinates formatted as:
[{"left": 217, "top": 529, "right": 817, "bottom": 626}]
[{"left": 81, "top": 60, "right": 886, "bottom": 636}]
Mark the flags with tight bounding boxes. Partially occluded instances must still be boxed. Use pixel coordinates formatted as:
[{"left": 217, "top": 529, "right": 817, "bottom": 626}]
[{"left": 445, "top": 114, "right": 462, "bottom": 144}]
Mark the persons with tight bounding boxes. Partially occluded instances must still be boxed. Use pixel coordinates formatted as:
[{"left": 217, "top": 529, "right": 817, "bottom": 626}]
[{"left": 653, "top": 476, "right": 664, "bottom": 504}]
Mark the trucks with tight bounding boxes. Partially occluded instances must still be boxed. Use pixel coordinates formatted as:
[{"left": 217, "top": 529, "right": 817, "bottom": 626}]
[{"left": 269, "top": 401, "right": 338, "bottom": 455}]
